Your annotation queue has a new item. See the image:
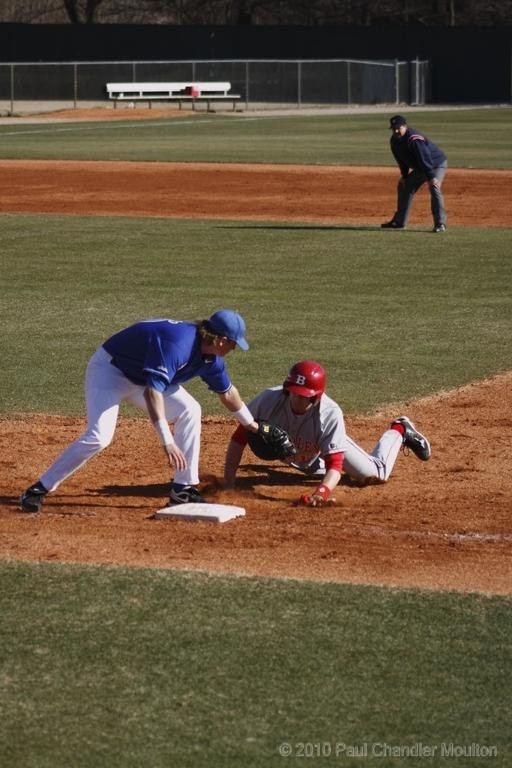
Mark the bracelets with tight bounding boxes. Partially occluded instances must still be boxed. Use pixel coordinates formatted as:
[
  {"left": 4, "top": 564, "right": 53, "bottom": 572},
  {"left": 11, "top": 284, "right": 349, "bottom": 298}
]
[
  {"left": 230, "top": 400, "right": 255, "bottom": 429},
  {"left": 313, "top": 482, "right": 330, "bottom": 504},
  {"left": 152, "top": 418, "right": 178, "bottom": 446}
]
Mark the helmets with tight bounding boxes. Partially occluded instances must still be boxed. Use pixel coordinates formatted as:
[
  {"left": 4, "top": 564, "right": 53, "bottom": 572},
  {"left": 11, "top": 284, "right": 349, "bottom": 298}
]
[{"left": 282, "top": 359, "right": 328, "bottom": 400}]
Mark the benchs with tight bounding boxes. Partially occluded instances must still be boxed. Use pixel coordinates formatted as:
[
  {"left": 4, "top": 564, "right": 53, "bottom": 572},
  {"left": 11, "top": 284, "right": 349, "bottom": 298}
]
[{"left": 99, "top": 76, "right": 241, "bottom": 114}]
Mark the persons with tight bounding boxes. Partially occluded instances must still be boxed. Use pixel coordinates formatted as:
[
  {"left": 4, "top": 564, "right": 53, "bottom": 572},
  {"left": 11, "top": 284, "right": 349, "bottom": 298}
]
[
  {"left": 19, "top": 305, "right": 299, "bottom": 514},
  {"left": 379, "top": 115, "right": 449, "bottom": 232},
  {"left": 203, "top": 358, "right": 431, "bottom": 507}
]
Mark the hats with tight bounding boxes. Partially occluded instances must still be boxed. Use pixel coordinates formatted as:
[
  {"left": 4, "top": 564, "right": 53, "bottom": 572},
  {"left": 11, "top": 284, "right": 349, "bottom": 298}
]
[
  {"left": 206, "top": 309, "right": 250, "bottom": 352},
  {"left": 387, "top": 115, "right": 407, "bottom": 130}
]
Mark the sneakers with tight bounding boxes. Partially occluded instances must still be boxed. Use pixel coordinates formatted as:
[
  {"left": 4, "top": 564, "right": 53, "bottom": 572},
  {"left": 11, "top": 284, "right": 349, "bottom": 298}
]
[
  {"left": 433, "top": 223, "right": 446, "bottom": 232},
  {"left": 19, "top": 486, "right": 47, "bottom": 513},
  {"left": 168, "top": 483, "right": 207, "bottom": 507},
  {"left": 389, "top": 415, "right": 431, "bottom": 461},
  {"left": 380, "top": 216, "right": 407, "bottom": 230}
]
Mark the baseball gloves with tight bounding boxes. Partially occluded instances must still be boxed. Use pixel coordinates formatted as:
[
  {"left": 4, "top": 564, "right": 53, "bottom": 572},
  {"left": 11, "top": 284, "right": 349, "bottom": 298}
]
[{"left": 248, "top": 421, "right": 297, "bottom": 459}]
